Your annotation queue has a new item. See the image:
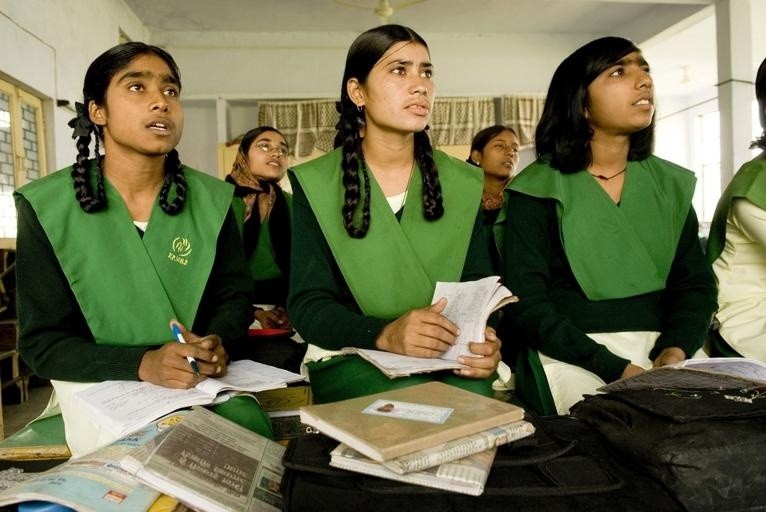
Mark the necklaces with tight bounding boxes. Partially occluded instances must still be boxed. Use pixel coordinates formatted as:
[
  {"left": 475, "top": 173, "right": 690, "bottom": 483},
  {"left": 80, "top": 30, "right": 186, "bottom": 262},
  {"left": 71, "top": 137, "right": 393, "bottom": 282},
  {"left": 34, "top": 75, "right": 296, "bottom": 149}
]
[{"left": 588, "top": 167, "right": 626, "bottom": 181}]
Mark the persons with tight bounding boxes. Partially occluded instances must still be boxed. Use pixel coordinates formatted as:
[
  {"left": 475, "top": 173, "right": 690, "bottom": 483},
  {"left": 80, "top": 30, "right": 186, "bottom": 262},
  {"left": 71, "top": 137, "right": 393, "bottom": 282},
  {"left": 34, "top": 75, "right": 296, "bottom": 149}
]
[
  {"left": 287, "top": 23, "right": 503, "bottom": 379},
  {"left": 499, "top": 36, "right": 719, "bottom": 386},
  {"left": 13, "top": 42, "right": 254, "bottom": 390},
  {"left": 704, "top": 57, "right": 766, "bottom": 365},
  {"left": 466, "top": 125, "right": 520, "bottom": 273},
  {"left": 224, "top": 127, "right": 294, "bottom": 330}
]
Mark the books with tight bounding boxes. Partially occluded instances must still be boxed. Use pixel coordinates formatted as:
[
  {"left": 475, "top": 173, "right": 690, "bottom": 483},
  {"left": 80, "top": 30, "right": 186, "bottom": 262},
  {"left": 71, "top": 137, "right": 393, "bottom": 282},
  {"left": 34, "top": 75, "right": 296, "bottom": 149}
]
[
  {"left": 299, "top": 382, "right": 535, "bottom": 497},
  {"left": 597, "top": 356, "right": 766, "bottom": 392},
  {"left": 341, "top": 274, "right": 520, "bottom": 380},
  {"left": 50, "top": 360, "right": 307, "bottom": 460},
  {"left": 1, "top": 407, "right": 286, "bottom": 511}
]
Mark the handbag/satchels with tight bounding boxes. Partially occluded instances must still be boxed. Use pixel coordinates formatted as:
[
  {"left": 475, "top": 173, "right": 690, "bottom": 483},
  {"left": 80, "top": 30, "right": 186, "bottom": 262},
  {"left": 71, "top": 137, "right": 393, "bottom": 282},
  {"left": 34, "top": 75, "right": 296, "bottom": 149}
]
[{"left": 564, "top": 387, "right": 765, "bottom": 512}]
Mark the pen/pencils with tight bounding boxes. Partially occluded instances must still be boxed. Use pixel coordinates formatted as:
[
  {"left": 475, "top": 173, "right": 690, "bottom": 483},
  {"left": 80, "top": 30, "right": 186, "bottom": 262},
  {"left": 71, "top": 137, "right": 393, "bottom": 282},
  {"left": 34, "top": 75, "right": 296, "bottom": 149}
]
[{"left": 174, "top": 324, "right": 199, "bottom": 373}]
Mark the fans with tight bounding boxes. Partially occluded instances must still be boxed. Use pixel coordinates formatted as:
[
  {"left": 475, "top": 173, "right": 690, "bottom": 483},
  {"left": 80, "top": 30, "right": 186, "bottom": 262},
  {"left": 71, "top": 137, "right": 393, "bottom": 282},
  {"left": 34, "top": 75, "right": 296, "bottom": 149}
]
[{"left": 333, "top": 0, "right": 424, "bottom": 26}]
